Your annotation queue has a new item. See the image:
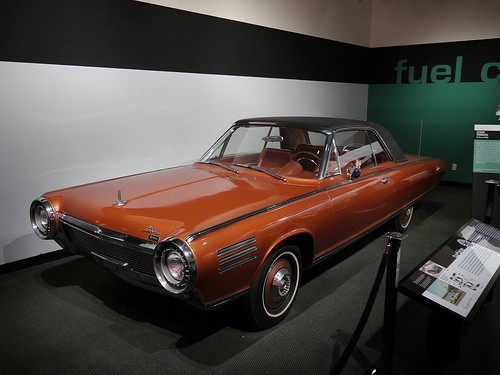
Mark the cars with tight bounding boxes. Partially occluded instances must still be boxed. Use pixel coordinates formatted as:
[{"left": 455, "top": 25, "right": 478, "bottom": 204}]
[{"left": 29, "top": 116, "right": 447, "bottom": 327}]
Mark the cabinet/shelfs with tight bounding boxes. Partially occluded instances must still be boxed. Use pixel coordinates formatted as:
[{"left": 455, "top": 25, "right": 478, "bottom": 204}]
[
  {"left": 397, "top": 217, "right": 500, "bottom": 365},
  {"left": 472, "top": 123, "right": 500, "bottom": 174}
]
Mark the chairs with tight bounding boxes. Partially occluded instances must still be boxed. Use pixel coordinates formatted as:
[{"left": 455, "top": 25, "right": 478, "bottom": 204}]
[
  {"left": 295, "top": 144, "right": 367, "bottom": 174},
  {"left": 258, "top": 147, "right": 292, "bottom": 172}
]
